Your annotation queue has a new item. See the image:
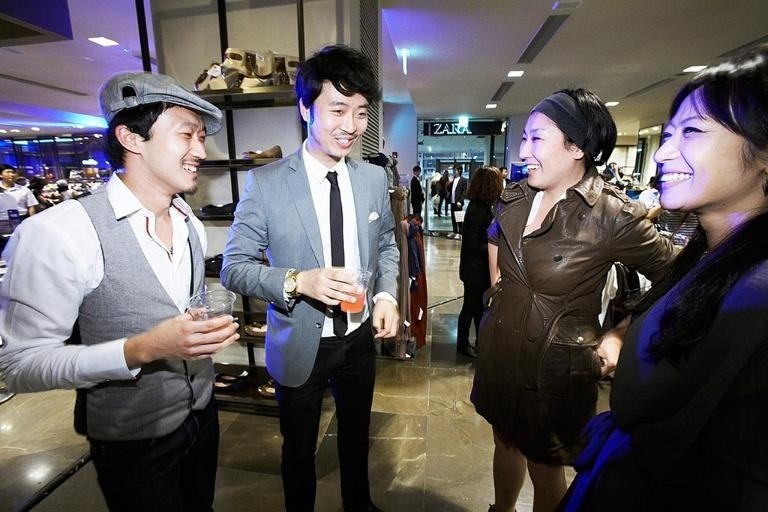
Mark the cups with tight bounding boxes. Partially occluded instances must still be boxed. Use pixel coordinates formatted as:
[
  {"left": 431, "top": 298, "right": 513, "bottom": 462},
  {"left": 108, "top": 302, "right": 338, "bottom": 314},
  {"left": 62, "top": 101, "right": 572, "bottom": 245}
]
[
  {"left": 337, "top": 262, "right": 370, "bottom": 317},
  {"left": 185, "top": 287, "right": 236, "bottom": 324}
]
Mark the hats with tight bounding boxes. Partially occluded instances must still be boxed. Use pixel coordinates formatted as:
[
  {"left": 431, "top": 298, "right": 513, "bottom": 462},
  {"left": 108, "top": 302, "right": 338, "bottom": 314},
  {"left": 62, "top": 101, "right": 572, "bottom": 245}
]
[{"left": 97, "top": 72, "right": 224, "bottom": 137}]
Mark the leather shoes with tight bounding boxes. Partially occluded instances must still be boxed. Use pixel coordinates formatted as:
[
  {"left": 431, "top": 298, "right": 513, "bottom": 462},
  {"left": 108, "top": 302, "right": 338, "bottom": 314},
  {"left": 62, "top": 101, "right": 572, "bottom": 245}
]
[
  {"left": 244, "top": 321, "right": 267, "bottom": 337},
  {"left": 257, "top": 384, "right": 276, "bottom": 397},
  {"left": 243, "top": 145, "right": 282, "bottom": 160},
  {"left": 199, "top": 203, "right": 236, "bottom": 214},
  {"left": 213, "top": 372, "right": 247, "bottom": 392}
]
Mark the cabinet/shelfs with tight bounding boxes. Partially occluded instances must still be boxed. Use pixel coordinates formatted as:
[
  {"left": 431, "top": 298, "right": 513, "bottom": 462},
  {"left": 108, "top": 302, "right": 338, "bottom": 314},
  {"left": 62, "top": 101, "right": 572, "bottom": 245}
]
[{"left": 131, "top": 2, "right": 349, "bottom": 415}]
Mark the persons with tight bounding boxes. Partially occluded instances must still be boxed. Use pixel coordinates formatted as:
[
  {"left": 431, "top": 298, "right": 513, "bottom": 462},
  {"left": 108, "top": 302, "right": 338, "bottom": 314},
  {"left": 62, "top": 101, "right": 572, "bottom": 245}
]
[
  {"left": 410, "top": 165, "right": 511, "bottom": 243},
  {"left": 555, "top": 54, "right": 768, "bottom": 511},
  {"left": 222, "top": 43, "right": 402, "bottom": 511},
  {"left": 467, "top": 85, "right": 680, "bottom": 512},
  {"left": 599, "top": 162, "right": 698, "bottom": 245},
  {"left": 0, "top": 159, "right": 115, "bottom": 259},
  {"left": 0, "top": 69, "right": 242, "bottom": 508},
  {"left": 455, "top": 164, "right": 505, "bottom": 360}
]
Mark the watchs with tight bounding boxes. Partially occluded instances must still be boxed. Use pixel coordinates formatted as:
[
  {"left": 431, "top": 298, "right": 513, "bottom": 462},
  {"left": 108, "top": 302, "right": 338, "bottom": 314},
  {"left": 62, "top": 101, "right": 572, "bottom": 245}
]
[{"left": 281, "top": 267, "right": 301, "bottom": 301}]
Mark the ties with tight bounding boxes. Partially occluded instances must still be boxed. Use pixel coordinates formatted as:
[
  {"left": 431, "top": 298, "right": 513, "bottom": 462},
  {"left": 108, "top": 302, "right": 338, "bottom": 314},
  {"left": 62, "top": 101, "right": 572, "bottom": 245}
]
[{"left": 325, "top": 171, "right": 347, "bottom": 338}]
